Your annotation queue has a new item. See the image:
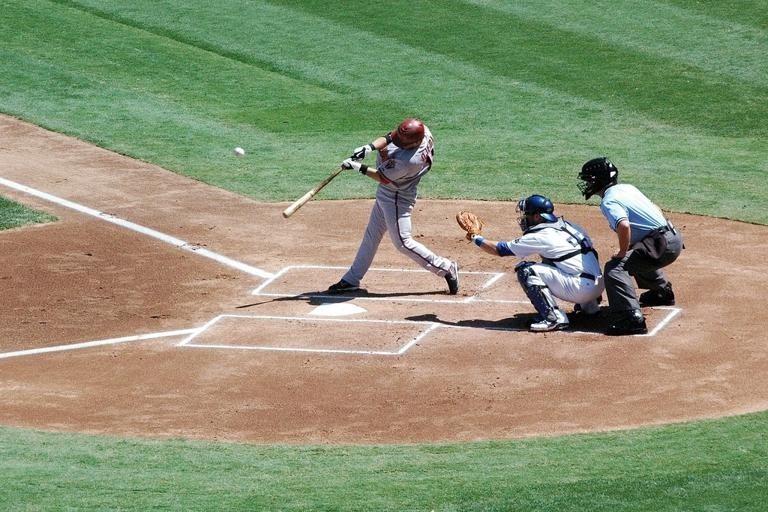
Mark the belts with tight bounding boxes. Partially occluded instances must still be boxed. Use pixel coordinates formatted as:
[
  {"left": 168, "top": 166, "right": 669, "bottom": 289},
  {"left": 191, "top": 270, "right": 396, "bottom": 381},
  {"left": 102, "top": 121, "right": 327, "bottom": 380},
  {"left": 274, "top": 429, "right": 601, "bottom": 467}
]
[{"left": 580, "top": 272, "right": 596, "bottom": 280}]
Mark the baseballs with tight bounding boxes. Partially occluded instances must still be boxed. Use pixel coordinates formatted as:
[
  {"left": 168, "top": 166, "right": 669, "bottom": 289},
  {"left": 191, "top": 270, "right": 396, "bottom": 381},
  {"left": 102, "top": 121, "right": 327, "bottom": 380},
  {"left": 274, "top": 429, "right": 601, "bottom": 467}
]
[{"left": 233, "top": 147, "right": 245, "bottom": 158}]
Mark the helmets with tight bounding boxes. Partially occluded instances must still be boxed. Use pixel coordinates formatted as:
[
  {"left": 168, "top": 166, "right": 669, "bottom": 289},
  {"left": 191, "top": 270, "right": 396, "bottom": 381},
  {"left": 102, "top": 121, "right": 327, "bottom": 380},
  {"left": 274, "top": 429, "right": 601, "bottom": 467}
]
[
  {"left": 391, "top": 119, "right": 425, "bottom": 147},
  {"left": 577, "top": 157, "right": 618, "bottom": 200},
  {"left": 516, "top": 195, "right": 558, "bottom": 231}
]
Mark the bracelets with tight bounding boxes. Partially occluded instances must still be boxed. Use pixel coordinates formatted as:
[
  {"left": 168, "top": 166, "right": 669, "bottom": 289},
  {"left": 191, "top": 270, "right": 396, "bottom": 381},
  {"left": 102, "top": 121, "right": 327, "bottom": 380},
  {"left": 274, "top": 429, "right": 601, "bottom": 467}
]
[
  {"left": 473, "top": 236, "right": 484, "bottom": 247},
  {"left": 369, "top": 143, "right": 376, "bottom": 151},
  {"left": 359, "top": 164, "right": 368, "bottom": 174}
]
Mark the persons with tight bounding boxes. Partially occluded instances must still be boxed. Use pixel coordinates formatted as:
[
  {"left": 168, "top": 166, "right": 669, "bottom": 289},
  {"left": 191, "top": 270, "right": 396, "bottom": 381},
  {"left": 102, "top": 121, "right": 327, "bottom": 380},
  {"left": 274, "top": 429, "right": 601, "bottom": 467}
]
[
  {"left": 577, "top": 158, "right": 686, "bottom": 336},
  {"left": 457, "top": 194, "right": 605, "bottom": 332},
  {"left": 326, "top": 118, "right": 459, "bottom": 295}
]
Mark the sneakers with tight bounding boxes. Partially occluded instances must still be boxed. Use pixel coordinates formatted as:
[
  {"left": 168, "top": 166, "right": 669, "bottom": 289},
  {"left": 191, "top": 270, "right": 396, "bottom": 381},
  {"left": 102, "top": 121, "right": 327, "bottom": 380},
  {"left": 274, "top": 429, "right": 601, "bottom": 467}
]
[
  {"left": 608, "top": 317, "right": 648, "bottom": 335},
  {"left": 329, "top": 279, "right": 360, "bottom": 293},
  {"left": 529, "top": 319, "right": 570, "bottom": 332},
  {"left": 640, "top": 287, "right": 674, "bottom": 305},
  {"left": 444, "top": 262, "right": 459, "bottom": 294}
]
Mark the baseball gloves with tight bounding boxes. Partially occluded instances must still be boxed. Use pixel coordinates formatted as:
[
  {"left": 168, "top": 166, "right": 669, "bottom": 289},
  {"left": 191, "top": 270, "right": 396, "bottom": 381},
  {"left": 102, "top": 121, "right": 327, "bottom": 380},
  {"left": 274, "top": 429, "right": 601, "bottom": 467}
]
[{"left": 456, "top": 211, "right": 485, "bottom": 240}]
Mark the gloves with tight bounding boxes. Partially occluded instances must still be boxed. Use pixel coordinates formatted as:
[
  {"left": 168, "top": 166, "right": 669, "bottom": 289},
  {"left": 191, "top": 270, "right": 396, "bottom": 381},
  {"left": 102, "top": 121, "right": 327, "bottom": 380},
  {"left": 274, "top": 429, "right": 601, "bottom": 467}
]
[
  {"left": 354, "top": 145, "right": 373, "bottom": 160},
  {"left": 343, "top": 159, "right": 362, "bottom": 172}
]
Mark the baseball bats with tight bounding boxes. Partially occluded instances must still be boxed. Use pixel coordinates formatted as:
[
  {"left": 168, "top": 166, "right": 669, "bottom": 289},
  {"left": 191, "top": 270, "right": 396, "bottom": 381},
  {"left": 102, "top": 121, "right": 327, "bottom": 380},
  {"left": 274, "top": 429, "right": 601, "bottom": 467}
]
[{"left": 283, "top": 156, "right": 359, "bottom": 220}]
